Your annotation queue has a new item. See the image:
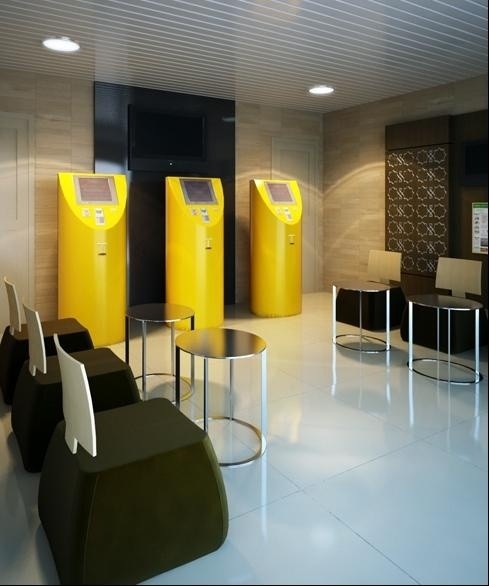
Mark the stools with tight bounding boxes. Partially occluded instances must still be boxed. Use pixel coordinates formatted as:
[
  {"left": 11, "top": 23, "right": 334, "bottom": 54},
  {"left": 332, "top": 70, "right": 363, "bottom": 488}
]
[
  {"left": 175, "top": 326, "right": 268, "bottom": 470},
  {"left": 405, "top": 292, "right": 484, "bottom": 385}
]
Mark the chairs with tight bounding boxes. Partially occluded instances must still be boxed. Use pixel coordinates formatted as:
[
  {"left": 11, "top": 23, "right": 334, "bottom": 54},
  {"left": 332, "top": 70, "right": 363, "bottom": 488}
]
[{"left": 331, "top": 248, "right": 486, "bottom": 356}]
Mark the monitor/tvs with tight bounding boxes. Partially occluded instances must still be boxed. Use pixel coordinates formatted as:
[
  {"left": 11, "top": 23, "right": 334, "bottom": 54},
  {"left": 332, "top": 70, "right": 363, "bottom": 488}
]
[
  {"left": 266, "top": 181, "right": 296, "bottom": 205},
  {"left": 128, "top": 103, "right": 208, "bottom": 175},
  {"left": 180, "top": 178, "right": 217, "bottom": 205},
  {"left": 74, "top": 175, "right": 118, "bottom": 205}
]
